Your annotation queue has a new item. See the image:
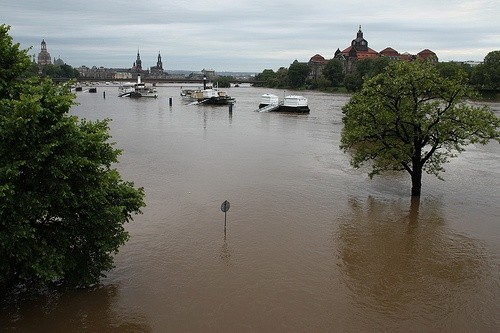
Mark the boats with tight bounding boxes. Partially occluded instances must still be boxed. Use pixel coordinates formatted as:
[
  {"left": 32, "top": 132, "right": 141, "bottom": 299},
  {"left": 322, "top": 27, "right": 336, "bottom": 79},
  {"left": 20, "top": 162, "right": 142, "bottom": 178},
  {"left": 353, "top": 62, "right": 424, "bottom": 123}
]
[
  {"left": 89, "top": 88, "right": 96, "bottom": 93},
  {"left": 75, "top": 87, "right": 82, "bottom": 91},
  {"left": 180, "top": 74, "right": 238, "bottom": 105},
  {"left": 258, "top": 94, "right": 310, "bottom": 114},
  {"left": 118, "top": 74, "right": 157, "bottom": 99}
]
